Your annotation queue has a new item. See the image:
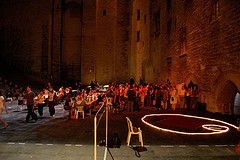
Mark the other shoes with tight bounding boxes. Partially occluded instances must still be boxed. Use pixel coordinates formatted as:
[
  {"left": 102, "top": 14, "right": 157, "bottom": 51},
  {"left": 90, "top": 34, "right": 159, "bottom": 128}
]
[{"left": 3, "top": 123, "right": 9, "bottom": 129}]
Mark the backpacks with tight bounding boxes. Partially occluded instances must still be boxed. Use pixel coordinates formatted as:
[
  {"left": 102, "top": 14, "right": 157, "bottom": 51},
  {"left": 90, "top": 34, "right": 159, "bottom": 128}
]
[{"left": 109, "top": 133, "right": 121, "bottom": 149}]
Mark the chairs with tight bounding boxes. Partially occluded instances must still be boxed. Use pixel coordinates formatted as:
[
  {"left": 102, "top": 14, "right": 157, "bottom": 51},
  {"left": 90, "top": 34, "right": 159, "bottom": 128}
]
[
  {"left": 75, "top": 108, "right": 85, "bottom": 119},
  {"left": 125, "top": 117, "right": 144, "bottom": 147}
]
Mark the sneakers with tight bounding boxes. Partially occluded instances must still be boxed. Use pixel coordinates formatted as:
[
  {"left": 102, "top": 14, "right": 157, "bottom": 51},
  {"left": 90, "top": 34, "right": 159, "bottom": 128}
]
[{"left": 23, "top": 115, "right": 55, "bottom": 123}]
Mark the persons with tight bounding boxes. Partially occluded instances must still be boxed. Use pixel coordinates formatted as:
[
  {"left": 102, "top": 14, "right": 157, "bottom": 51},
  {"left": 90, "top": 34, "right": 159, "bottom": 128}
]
[
  {"left": 22, "top": 86, "right": 38, "bottom": 122},
  {"left": 0, "top": 91, "right": 9, "bottom": 129},
  {"left": 0, "top": 78, "right": 198, "bottom": 117}
]
